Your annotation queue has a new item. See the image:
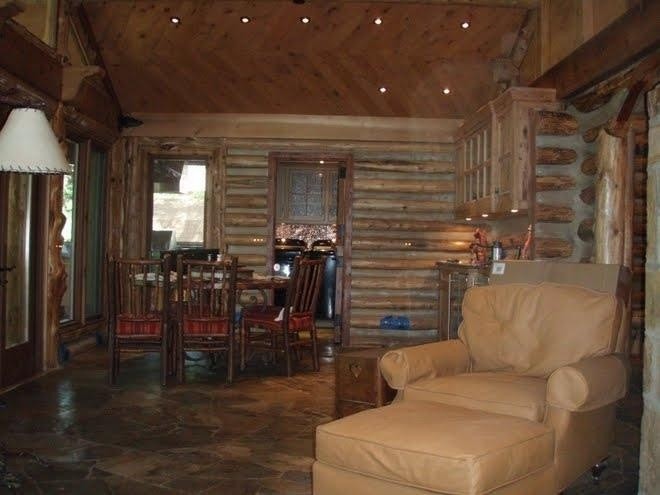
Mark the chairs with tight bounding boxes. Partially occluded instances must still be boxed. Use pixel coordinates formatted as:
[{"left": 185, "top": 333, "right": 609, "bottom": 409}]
[
  {"left": 107, "top": 254, "right": 178, "bottom": 386},
  {"left": 171, "top": 254, "right": 239, "bottom": 385},
  {"left": 238, "top": 256, "right": 327, "bottom": 378},
  {"left": 161, "top": 249, "right": 219, "bottom": 273}
]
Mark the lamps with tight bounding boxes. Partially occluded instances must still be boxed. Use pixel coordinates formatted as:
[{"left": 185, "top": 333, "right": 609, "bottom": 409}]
[{"left": 0, "top": 106, "right": 72, "bottom": 174}]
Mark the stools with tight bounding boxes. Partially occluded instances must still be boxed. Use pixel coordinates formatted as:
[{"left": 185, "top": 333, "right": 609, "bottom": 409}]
[{"left": 312, "top": 399, "right": 556, "bottom": 494}]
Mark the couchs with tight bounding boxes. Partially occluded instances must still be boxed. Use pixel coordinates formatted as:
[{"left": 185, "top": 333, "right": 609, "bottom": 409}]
[{"left": 312, "top": 260, "right": 632, "bottom": 494}]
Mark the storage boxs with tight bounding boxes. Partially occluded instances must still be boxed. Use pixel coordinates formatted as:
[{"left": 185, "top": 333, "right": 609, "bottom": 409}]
[{"left": 333, "top": 346, "right": 415, "bottom": 417}]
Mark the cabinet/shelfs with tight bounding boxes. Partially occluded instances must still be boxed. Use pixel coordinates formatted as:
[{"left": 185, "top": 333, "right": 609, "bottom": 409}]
[
  {"left": 437, "top": 262, "right": 491, "bottom": 342},
  {"left": 452, "top": 86, "right": 556, "bottom": 220}
]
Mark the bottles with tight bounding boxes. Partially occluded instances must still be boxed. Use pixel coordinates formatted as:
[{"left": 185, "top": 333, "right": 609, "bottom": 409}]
[{"left": 492, "top": 242, "right": 502, "bottom": 262}]
